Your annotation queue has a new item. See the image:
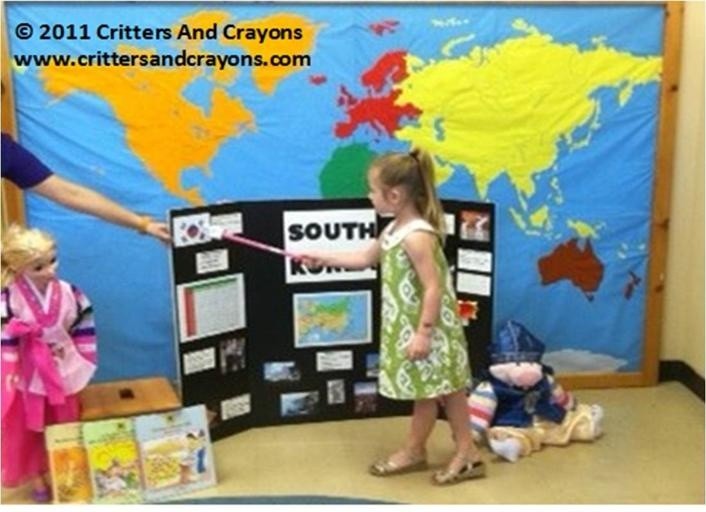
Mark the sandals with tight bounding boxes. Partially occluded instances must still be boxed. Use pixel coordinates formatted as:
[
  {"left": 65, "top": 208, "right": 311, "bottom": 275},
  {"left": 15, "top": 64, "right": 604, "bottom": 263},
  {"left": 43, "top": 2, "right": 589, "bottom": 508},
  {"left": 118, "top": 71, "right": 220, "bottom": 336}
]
[
  {"left": 430, "top": 451, "right": 488, "bottom": 488},
  {"left": 368, "top": 443, "right": 429, "bottom": 478}
]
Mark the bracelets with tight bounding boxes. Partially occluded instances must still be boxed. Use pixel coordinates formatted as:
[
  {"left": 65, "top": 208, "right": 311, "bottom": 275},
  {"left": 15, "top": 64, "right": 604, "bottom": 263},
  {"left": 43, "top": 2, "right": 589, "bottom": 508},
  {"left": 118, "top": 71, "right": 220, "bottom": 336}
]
[
  {"left": 137, "top": 212, "right": 151, "bottom": 236},
  {"left": 420, "top": 320, "right": 432, "bottom": 328}
]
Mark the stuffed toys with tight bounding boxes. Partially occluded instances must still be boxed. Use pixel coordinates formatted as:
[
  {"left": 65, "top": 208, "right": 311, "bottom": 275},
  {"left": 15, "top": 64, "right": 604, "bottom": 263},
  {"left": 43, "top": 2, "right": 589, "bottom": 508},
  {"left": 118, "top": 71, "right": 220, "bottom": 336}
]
[{"left": 467, "top": 318, "right": 605, "bottom": 462}]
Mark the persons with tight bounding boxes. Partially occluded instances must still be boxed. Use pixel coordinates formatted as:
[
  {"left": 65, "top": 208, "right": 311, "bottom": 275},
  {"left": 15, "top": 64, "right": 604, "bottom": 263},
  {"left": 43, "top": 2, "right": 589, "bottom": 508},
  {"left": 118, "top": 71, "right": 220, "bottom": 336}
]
[
  {"left": 293, "top": 144, "right": 486, "bottom": 483},
  {"left": 0, "top": 80, "right": 176, "bottom": 249},
  {"left": 0, "top": 224, "right": 96, "bottom": 504}
]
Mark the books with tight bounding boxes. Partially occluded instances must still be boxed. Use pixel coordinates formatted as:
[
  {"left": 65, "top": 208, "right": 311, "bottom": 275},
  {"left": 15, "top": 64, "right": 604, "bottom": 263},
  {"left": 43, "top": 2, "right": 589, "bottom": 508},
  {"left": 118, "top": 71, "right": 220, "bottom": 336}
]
[
  {"left": 44, "top": 421, "right": 97, "bottom": 504},
  {"left": 81, "top": 415, "right": 144, "bottom": 504},
  {"left": 134, "top": 403, "right": 218, "bottom": 502}
]
